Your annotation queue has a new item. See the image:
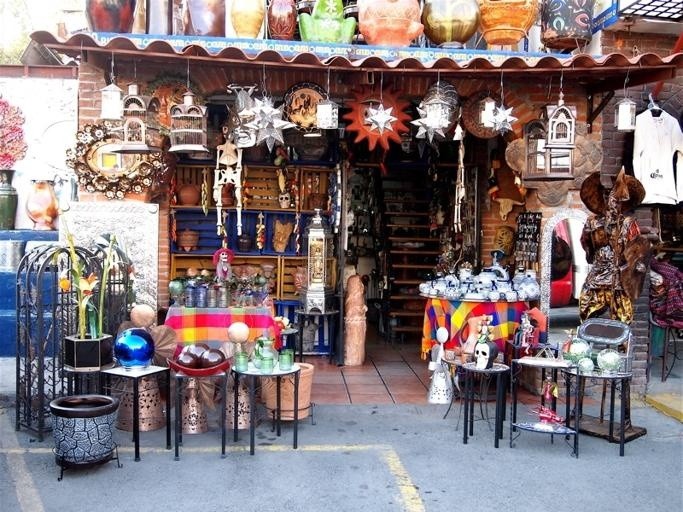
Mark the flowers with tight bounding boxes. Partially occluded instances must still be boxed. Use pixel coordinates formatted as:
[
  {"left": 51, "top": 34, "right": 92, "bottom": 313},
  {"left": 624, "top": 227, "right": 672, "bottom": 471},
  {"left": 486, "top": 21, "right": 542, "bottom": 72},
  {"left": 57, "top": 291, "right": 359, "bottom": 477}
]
[
  {"left": 0, "top": 92, "right": 28, "bottom": 169},
  {"left": 47, "top": 206, "right": 129, "bottom": 341}
]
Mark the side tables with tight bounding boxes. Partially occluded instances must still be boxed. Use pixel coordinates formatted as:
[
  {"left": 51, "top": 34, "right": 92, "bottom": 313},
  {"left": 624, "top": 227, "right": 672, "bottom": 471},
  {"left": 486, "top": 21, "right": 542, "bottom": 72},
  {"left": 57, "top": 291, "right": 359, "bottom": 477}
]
[
  {"left": 296, "top": 307, "right": 339, "bottom": 365},
  {"left": 441, "top": 339, "right": 633, "bottom": 459},
  {"left": 100, "top": 358, "right": 299, "bottom": 463}
]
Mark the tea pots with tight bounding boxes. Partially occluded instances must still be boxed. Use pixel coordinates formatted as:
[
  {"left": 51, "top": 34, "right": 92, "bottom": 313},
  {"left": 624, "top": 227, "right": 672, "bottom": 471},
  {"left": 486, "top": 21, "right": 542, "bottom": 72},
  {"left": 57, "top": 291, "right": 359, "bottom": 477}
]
[
  {"left": 252, "top": 336, "right": 278, "bottom": 369},
  {"left": 492, "top": 279, "right": 514, "bottom": 299},
  {"left": 431, "top": 277, "right": 474, "bottom": 299}
]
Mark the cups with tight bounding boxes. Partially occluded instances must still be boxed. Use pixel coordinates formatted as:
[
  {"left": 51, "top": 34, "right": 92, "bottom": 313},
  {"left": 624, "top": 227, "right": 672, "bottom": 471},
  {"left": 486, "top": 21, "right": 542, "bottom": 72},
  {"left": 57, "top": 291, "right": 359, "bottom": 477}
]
[
  {"left": 234, "top": 350, "right": 294, "bottom": 374},
  {"left": 489, "top": 291, "right": 525, "bottom": 303},
  {"left": 419, "top": 283, "right": 438, "bottom": 295}
]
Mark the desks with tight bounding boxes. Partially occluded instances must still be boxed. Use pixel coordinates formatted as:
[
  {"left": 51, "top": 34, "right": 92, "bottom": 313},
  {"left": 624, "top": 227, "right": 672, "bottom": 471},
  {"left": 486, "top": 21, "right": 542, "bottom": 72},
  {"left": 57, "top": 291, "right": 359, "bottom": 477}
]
[
  {"left": 416, "top": 294, "right": 539, "bottom": 358},
  {"left": 167, "top": 302, "right": 273, "bottom": 347}
]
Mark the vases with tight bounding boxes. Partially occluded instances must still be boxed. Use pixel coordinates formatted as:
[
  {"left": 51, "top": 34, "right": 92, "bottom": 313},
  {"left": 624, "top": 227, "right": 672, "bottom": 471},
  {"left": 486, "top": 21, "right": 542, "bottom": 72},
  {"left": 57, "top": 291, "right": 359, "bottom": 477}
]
[
  {"left": 0, "top": 168, "right": 59, "bottom": 231},
  {"left": 265, "top": 363, "right": 314, "bottom": 421},
  {"left": 50, "top": 331, "right": 120, "bottom": 468},
  {"left": 176, "top": 184, "right": 252, "bottom": 252}
]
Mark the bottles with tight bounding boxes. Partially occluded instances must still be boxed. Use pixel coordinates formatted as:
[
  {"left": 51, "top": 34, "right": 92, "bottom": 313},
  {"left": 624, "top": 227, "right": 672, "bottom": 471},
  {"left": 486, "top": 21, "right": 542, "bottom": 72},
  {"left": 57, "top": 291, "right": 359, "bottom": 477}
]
[
  {"left": 0, "top": 169, "right": 60, "bottom": 230},
  {"left": 178, "top": 185, "right": 200, "bottom": 206},
  {"left": 238, "top": 233, "right": 251, "bottom": 252}
]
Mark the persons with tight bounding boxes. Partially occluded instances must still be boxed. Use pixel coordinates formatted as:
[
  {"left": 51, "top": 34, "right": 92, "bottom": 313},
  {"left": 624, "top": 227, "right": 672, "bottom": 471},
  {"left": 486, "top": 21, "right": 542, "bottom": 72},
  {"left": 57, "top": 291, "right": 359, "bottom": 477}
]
[{"left": 570, "top": 172, "right": 645, "bottom": 436}]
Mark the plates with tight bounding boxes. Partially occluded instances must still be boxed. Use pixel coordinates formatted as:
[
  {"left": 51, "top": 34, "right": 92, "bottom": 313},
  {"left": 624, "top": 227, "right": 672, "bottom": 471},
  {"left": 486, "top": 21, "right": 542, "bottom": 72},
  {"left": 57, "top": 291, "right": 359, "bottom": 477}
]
[{"left": 283, "top": 81, "right": 330, "bottom": 132}]
